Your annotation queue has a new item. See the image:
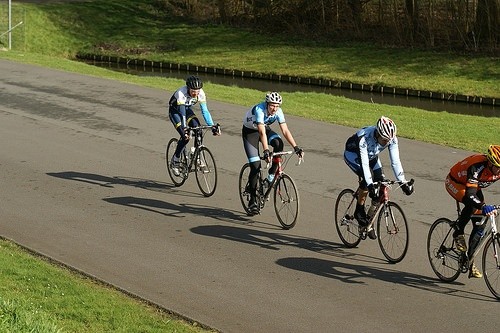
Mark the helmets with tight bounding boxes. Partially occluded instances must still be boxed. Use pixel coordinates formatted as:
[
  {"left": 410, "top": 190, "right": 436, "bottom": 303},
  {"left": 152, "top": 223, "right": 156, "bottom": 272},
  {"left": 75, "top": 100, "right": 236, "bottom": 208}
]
[
  {"left": 376, "top": 116, "right": 396, "bottom": 141},
  {"left": 266, "top": 92, "right": 282, "bottom": 105},
  {"left": 186, "top": 76, "right": 203, "bottom": 89},
  {"left": 487, "top": 145, "right": 500, "bottom": 167}
]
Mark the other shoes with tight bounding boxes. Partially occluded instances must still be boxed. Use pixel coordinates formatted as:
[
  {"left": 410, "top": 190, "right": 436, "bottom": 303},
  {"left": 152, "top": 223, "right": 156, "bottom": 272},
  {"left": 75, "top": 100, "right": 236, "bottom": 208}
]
[
  {"left": 248, "top": 202, "right": 259, "bottom": 214},
  {"left": 368, "top": 228, "right": 377, "bottom": 239},
  {"left": 267, "top": 172, "right": 276, "bottom": 187},
  {"left": 354, "top": 210, "right": 369, "bottom": 226},
  {"left": 171, "top": 156, "right": 180, "bottom": 176}
]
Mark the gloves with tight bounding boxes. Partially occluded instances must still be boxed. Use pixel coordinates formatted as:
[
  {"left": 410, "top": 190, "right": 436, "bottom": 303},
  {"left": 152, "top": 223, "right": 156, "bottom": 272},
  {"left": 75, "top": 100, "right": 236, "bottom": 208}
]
[
  {"left": 367, "top": 184, "right": 378, "bottom": 198},
  {"left": 399, "top": 182, "right": 415, "bottom": 196}
]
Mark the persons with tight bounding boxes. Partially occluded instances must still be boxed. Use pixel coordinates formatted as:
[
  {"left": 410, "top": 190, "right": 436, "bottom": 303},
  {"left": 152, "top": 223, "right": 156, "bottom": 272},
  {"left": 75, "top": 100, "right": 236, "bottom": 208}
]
[
  {"left": 445, "top": 145, "right": 500, "bottom": 278},
  {"left": 167, "top": 76, "right": 222, "bottom": 176},
  {"left": 242, "top": 92, "right": 304, "bottom": 214},
  {"left": 343, "top": 116, "right": 414, "bottom": 239}
]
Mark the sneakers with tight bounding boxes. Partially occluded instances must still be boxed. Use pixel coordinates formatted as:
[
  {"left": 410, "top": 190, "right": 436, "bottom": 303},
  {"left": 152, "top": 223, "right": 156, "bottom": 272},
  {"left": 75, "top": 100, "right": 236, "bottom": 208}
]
[
  {"left": 469, "top": 262, "right": 482, "bottom": 278},
  {"left": 452, "top": 231, "right": 467, "bottom": 251}
]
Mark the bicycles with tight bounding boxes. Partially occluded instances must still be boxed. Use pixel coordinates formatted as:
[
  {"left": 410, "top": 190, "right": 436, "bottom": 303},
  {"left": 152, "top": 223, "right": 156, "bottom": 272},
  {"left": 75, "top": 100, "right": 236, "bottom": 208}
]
[
  {"left": 166, "top": 122, "right": 219, "bottom": 197},
  {"left": 335, "top": 161, "right": 414, "bottom": 263},
  {"left": 239, "top": 142, "right": 303, "bottom": 230},
  {"left": 427, "top": 198, "right": 500, "bottom": 301}
]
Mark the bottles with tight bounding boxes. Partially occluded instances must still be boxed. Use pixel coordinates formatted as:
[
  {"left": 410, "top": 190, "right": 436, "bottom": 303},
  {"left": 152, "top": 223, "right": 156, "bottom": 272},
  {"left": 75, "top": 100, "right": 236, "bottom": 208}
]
[
  {"left": 470, "top": 230, "right": 482, "bottom": 247},
  {"left": 265, "top": 174, "right": 274, "bottom": 187},
  {"left": 367, "top": 201, "right": 379, "bottom": 220},
  {"left": 188, "top": 147, "right": 196, "bottom": 160}
]
